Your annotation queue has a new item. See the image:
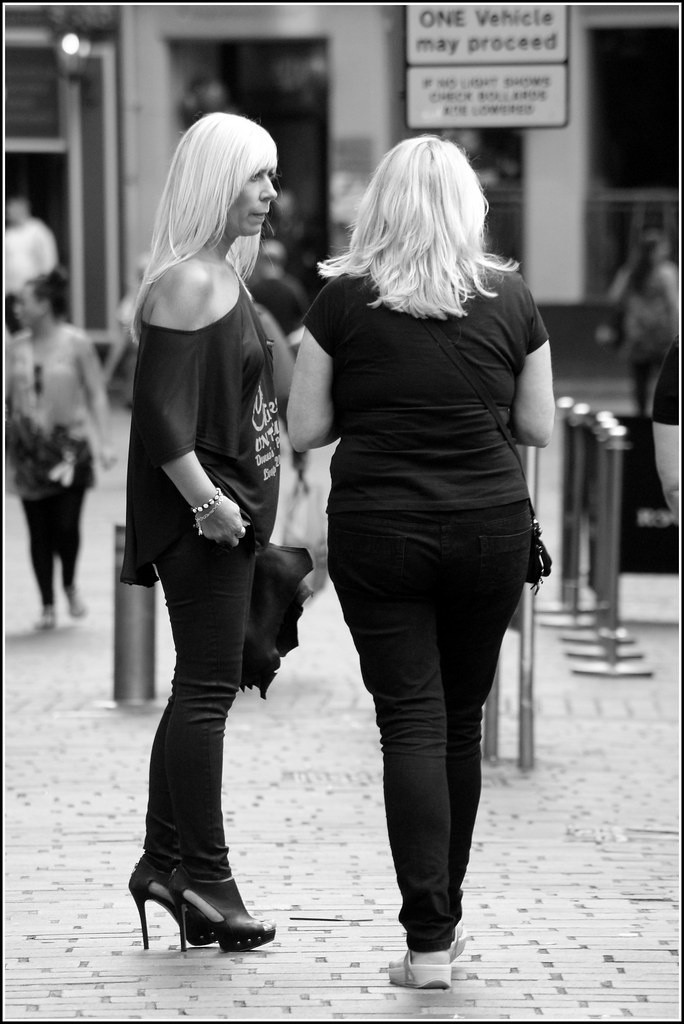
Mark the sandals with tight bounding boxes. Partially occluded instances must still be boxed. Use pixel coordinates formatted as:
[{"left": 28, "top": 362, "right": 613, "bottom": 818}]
[{"left": 388, "top": 920, "right": 467, "bottom": 989}]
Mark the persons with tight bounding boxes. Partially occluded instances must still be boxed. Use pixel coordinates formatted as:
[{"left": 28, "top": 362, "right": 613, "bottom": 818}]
[
  {"left": 6, "top": 194, "right": 62, "bottom": 344},
  {"left": 604, "top": 227, "right": 679, "bottom": 420},
  {"left": 115, "top": 108, "right": 284, "bottom": 957},
  {"left": 5, "top": 266, "right": 120, "bottom": 632},
  {"left": 285, "top": 133, "right": 557, "bottom": 993}
]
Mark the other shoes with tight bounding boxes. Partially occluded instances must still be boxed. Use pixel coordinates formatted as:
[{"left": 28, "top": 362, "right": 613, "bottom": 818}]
[
  {"left": 36, "top": 608, "right": 55, "bottom": 630},
  {"left": 66, "top": 587, "right": 88, "bottom": 617}
]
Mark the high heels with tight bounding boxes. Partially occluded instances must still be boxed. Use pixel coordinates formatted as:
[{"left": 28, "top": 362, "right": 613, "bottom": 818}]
[
  {"left": 128, "top": 853, "right": 219, "bottom": 950},
  {"left": 169, "top": 864, "right": 275, "bottom": 952}
]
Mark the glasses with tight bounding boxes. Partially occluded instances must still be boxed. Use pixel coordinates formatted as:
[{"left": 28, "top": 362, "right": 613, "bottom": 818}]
[{"left": 33, "top": 365, "right": 43, "bottom": 395}]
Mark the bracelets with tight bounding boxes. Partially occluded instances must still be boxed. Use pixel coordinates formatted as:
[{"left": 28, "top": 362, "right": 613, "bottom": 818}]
[{"left": 191, "top": 487, "right": 224, "bottom": 536}]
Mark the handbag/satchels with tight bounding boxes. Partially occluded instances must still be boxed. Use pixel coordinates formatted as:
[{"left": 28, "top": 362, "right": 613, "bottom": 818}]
[
  {"left": 602, "top": 307, "right": 624, "bottom": 350},
  {"left": 526, "top": 518, "right": 552, "bottom": 592},
  {"left": 44, "top": 421, "right": 88, "bottom": 491}
]
